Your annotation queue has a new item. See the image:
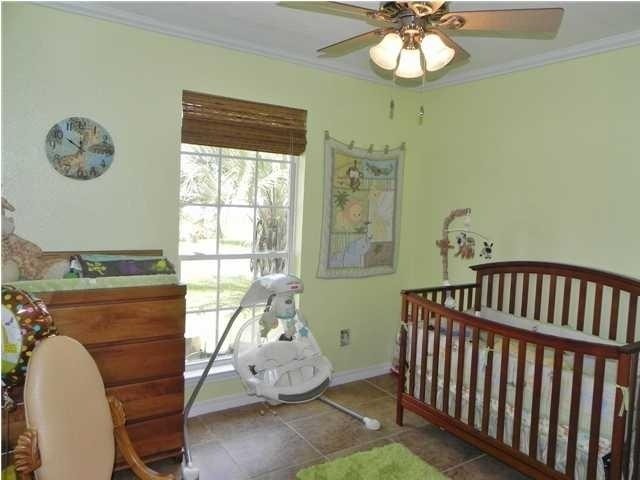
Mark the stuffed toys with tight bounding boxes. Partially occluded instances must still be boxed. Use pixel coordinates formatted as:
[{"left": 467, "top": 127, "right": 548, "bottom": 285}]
[
  {"left": 259, "top": 312, "right": 309, "bottom": 342},
  {"left": 435, "top": 233, "right": 494, "bottom": 261},
  {"left": 1, "top": 196, "right": 69, "bottom": 281}
]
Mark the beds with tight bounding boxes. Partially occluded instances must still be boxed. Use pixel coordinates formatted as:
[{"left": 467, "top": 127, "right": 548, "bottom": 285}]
[
  {"left": 1, "top": 249, "right": 185, "bottom": 470},
  {"left": 397, "top": 261, "right": 640, "bottom": 480}
]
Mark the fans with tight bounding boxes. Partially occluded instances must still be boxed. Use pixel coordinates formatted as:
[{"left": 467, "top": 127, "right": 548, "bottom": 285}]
[{"left": 278, "top": 1, "right": 565, "bottom": 56}]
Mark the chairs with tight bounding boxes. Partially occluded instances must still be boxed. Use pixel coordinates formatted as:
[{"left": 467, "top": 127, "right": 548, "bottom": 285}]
[{"left": 15, "top": 335, "right": 200, "bottom": 480}]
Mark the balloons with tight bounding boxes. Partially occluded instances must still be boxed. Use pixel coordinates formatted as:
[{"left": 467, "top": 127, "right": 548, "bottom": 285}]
[{"left": 1, "top": 284, "right": 57, "bottom": 412}]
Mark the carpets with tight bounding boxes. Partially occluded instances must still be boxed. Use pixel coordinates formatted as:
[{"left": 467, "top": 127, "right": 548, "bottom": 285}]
[{"left": 297, "top": 444, "right": 451, "bottom": 480}]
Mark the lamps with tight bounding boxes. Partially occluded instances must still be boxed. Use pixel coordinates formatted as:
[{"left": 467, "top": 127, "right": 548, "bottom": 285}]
[{"left": 370, "top": 13, "right": 454, "bottom": 125}]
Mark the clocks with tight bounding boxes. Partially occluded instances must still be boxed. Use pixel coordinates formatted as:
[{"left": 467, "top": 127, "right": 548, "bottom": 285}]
[{"left": 46, "top": 118, "right": 115, "bottom": 180}]
